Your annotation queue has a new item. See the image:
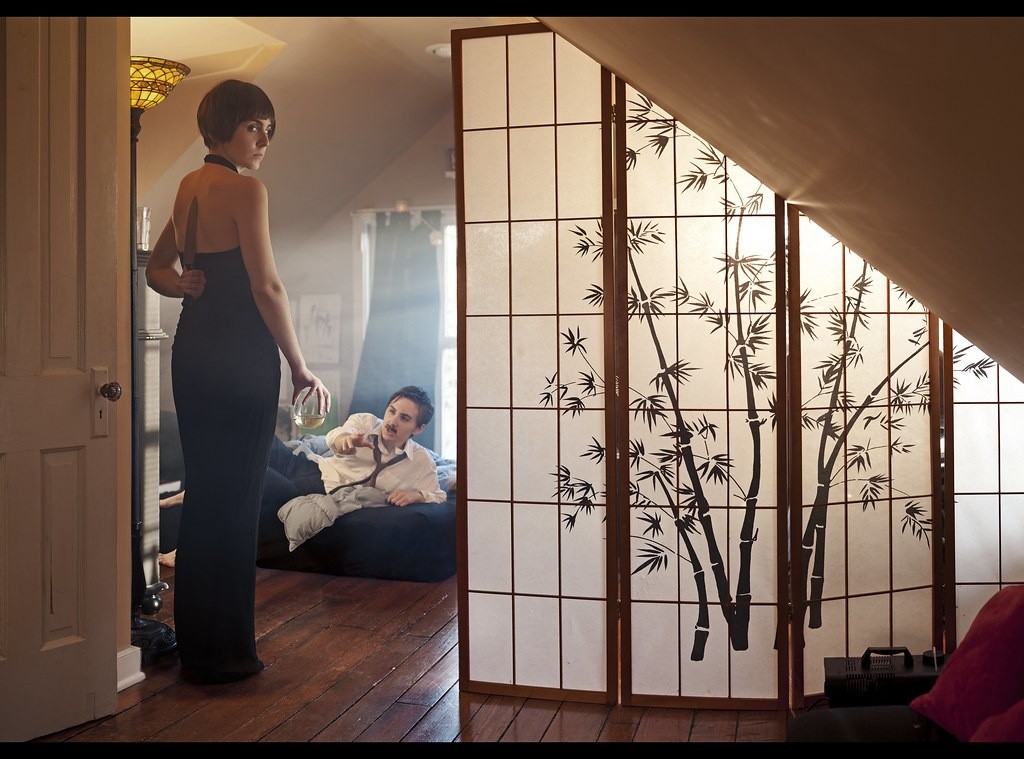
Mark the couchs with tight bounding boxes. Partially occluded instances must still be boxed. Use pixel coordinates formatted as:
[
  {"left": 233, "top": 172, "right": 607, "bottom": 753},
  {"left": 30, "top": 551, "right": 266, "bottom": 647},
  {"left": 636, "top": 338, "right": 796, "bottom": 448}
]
[{"left": 784, "top": 583, "right": 1024, "bottom": 745}]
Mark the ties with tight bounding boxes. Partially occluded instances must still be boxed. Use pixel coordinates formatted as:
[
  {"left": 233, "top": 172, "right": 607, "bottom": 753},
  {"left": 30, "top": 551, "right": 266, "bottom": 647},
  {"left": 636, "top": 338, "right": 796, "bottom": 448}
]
[{"left": 329, "top": 435, "right": 407, "bottom": 495}]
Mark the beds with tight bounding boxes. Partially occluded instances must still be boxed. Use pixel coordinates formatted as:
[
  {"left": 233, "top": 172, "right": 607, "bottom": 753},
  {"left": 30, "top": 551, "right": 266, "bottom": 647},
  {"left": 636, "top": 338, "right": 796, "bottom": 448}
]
[{"left": 157, "top": 435, "right": 459, "bottom": 582}]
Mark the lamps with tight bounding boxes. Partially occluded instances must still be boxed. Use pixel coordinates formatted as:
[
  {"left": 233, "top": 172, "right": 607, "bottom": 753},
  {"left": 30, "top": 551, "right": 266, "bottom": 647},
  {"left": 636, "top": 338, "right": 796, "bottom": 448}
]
[{"left": 129, "top": 54, "right": 192, "bottom": 673}]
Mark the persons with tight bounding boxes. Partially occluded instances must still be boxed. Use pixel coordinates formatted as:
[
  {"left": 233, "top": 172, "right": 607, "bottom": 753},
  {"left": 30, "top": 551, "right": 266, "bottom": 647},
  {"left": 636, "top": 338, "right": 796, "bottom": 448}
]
[
  {"left": 146, "top": 80, "right": 330, "bottom": 679},
  {"left": 159, "top": 385, "right": 447, "bottom": 568}
]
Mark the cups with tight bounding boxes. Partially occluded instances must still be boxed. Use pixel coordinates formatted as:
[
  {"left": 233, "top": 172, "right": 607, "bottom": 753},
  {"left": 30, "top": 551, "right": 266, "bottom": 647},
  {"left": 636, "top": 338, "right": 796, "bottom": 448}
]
[
  {"left": 137, "top": 206, "right": 151, "bottom": 251},
  {"left": 293, "top": 387, "right": 326, "bottom": 429}
]
